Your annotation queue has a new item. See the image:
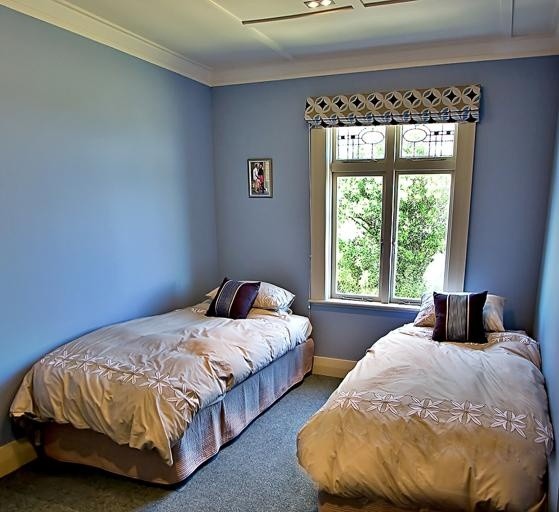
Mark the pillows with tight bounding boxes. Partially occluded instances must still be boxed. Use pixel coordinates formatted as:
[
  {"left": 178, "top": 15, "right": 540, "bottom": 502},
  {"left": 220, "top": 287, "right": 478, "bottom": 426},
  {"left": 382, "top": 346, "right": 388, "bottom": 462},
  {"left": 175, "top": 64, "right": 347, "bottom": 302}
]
[
  {"left": 415, "top": 294, "right": 505, "bottom": 333},
  {"left": 431, "top": 293, "right": 488, "bottom": 344},
  {"left": 206, "top": 280, "right": 261, "bottom": 317},
  {"left": 207, "top": 282, "right": 293, "bottom": 308}
]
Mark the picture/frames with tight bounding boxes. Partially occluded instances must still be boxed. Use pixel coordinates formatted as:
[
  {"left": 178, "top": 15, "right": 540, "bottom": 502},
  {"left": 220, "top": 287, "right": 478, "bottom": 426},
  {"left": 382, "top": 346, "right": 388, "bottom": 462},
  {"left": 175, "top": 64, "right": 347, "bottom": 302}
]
[{"left": 248, "top": 159, "right": 272, "bottom": 197}]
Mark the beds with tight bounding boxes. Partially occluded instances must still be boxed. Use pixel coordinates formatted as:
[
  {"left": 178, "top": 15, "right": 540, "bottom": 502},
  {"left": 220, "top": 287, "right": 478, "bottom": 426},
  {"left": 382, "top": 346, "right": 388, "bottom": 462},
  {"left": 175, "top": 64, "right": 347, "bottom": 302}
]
[
  {"left": 9, "top": 278, "right": 315, "bottom": 486},
  {"left": 297, "top": 323, "right": 554, "bottom": 512}
]
